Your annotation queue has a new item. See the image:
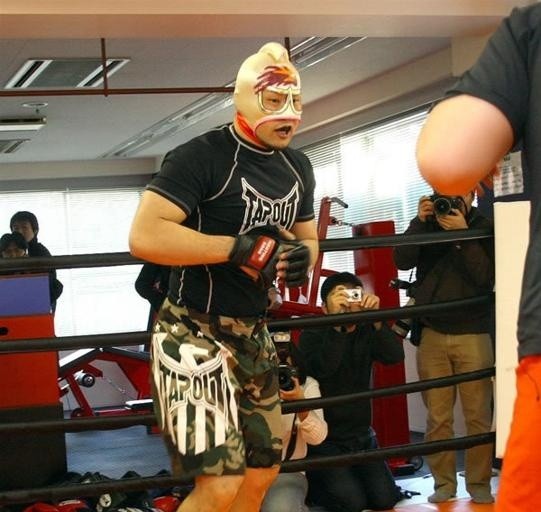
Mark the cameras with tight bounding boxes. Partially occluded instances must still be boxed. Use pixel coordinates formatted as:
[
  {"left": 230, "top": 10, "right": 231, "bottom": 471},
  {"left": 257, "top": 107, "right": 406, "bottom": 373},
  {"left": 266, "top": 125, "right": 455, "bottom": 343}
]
[
  {"left": 341, "top": 288, "right": 361, "bottom": 303},
  {"left": 271, "top": 330, "right": 299, "bottom": 391},
  {"left": 427, "top": 194, "right": 462, "bottom": 216},
  {"left": 388, "top": 280, "right": 415, "bottom": 338}
]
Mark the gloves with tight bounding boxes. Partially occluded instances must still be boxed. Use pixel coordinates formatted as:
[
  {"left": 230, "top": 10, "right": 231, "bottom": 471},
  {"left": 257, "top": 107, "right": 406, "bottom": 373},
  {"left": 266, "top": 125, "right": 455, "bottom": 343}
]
[
  {"left": 19, "top": 469, "right": 196, "bottom": 512},
  {"left": 285, "top": 239, "right": 309, "bottom": 289},
  {"left": 226, "top": 224, "right": 284, "bottom": 292}
]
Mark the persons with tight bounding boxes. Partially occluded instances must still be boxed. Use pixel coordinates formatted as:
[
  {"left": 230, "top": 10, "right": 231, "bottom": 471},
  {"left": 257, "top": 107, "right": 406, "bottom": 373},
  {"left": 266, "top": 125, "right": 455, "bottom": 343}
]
[
  {"left": 393, "top": 187, "right": 494, "bottom": 504},
  {"left": 0, "top": 211, "right": 63, "bottom": 317},
  {"left": 418, "top": 4, "right": 541, "bottom": 512},
  {"left": 129, "top": 44, "right": 319, "bottom": 512},
  {"left": 259, "top": 334, "right": 327, "bottom": 511},
  {"left": 296, "top": 272, "right": 405, "bottom": 511}
]
